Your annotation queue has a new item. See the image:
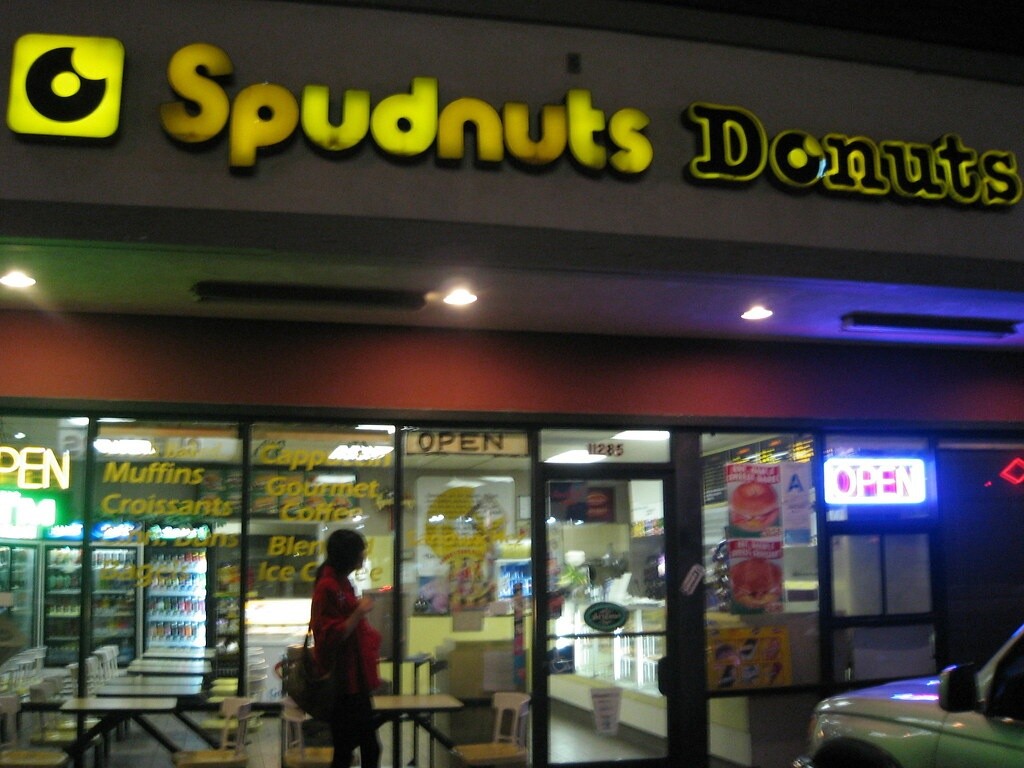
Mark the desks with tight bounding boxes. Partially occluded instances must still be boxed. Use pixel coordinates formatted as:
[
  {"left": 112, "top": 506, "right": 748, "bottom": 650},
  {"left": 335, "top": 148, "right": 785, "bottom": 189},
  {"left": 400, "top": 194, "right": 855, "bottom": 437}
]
[
  {"left": 60, "top": 644, "right": 217, "bottom": 765},
  {"left": 372, "top": 692, "right": 464, "bottom": 768}
]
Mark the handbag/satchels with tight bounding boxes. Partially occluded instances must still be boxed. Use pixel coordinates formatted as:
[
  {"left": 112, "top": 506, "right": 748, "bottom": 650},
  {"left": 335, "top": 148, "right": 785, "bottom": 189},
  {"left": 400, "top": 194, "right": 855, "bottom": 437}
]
[{"left": 286, "top": 647, "right": 347, "bottom": 724}]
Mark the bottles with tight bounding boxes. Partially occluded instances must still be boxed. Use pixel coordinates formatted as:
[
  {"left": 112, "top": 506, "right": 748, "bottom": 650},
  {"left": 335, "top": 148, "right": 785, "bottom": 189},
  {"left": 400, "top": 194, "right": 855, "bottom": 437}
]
[{"left": 46, "top": 545, "right": 205, "bottom": 654}]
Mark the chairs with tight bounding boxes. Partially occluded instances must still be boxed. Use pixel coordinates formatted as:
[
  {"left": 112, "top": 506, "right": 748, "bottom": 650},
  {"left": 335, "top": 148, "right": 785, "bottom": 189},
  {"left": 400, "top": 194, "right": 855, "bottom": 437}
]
[
  {"left": 282, "top": 695, "right": 358, "bottom": 768},
  {"left": 169, "top": 645, "right": 267, "bottom": 768},
  {"left": 385, "top": 652, "right": 437, "bottom": 768},
  {"left": 451, "top": 690, "right": 532, "bottom": 768},
  {"left": 0, "top": 646, "right": 129, "bottom": 768}
]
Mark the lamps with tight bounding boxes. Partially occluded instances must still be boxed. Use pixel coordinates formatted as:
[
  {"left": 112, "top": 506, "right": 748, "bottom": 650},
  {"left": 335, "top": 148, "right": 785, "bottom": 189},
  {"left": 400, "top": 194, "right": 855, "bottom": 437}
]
[{"left": 842, "top": 310, "right": 1020, "bottom": 339}]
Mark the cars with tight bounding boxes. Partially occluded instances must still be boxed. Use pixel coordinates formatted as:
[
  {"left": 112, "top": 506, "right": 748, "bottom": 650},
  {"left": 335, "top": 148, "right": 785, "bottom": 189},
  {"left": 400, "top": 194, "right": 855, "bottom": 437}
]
[{"left": 791, "top": 622, "right": 1024, "bottom": 768}]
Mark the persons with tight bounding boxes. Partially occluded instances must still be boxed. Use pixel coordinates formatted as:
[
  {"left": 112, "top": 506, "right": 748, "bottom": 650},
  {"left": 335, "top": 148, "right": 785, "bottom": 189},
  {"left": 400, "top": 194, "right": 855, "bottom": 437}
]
[{"left": 310, "top": 529, "right": 384, "bottom": 768}]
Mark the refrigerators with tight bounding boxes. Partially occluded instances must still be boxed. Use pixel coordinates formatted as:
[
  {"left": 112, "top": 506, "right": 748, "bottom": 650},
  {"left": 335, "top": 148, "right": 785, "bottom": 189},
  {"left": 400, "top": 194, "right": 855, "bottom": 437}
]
[{"left": 39, "top": 520, "right": 218, "bottom": 668}]
